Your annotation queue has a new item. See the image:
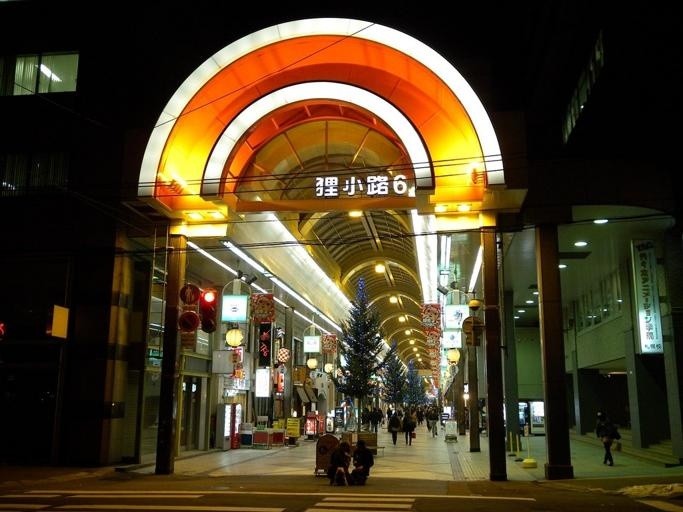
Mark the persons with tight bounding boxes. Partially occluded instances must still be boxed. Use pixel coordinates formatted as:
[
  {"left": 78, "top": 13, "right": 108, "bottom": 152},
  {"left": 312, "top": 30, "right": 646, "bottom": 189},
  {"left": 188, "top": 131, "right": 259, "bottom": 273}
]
[
  {"left": 326, "top": 442, "right": 352, "bottom": 485},
  {"left": 595, "top": 410, "right": 621, "bottom": 466},
  {"left": 361, "top": 403, "right": 443, "bottom": 446},
  {"left": 351, "top": 439, "right": 374, "bottom": 485}
]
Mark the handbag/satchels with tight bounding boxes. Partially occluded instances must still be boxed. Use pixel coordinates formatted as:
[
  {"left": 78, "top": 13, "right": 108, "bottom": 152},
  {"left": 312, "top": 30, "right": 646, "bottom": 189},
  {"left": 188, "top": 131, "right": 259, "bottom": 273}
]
[
  {"left": 617, "top": 443, "right": 621, "bottom": 451},
  {"left": 412, "top": 432, "right": 416, "bottom": 438}
]
